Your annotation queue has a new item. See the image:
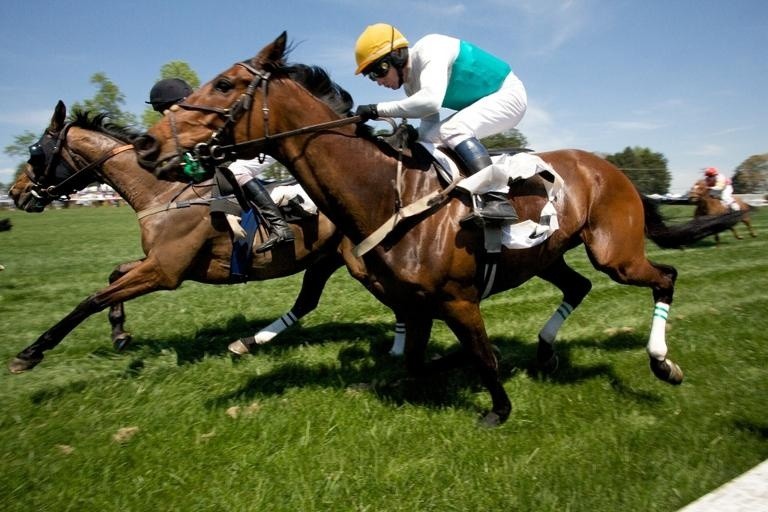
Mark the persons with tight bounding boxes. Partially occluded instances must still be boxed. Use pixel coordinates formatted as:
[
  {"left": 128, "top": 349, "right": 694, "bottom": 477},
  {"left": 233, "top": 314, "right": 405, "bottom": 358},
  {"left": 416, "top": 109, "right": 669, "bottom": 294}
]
[
  {"left": 143, "top": 78, "right": 295, "bottom": 254},
  {"left": 354, "top": 23, "right": 528, "bottom": 231},
  {"left": 702, "top": 168, "right": 740, "bottom": 211}
]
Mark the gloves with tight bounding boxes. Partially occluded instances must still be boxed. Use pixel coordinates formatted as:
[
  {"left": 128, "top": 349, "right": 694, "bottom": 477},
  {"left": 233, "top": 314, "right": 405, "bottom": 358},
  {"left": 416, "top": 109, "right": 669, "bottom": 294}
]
[
  {"left": 355, "top": 104, "right": 378, "bottom": 123},
  {"left": 399, "top": 124, "right": 418, "bottom": 143}
]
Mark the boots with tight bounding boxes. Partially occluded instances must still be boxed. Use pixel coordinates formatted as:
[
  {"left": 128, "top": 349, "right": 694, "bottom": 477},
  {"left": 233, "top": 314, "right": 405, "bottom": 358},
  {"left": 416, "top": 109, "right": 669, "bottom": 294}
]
[
  {"left": 454, "top": 137, "right": 519, "bottom": 229},
  {"left": 242, "top": 178, "right": 296, "bottom": 253}
]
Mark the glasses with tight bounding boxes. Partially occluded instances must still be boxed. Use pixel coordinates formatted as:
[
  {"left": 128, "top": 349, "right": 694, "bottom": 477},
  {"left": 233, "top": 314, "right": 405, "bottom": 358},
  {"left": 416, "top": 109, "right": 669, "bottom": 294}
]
[{"left": 370, "top": 61, "right": 389, "bottom": 80}]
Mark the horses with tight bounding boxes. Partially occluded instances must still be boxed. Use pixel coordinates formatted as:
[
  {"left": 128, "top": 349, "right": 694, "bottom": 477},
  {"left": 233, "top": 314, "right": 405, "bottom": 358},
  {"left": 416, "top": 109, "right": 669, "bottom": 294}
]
[
  {"left": 687, "top": 179, "right": 760, "bottom": 241},
  {"left": 8, "top": 99, "right": 407, "bottom": 374},
  {"left": 132, "top": 30, "right": 751, "bottom": 430}
]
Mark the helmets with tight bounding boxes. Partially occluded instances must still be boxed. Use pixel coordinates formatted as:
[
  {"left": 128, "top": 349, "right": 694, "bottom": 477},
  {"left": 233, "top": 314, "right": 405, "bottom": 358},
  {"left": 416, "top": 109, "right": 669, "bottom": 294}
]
[
  {"left": 704, "top": 168, "right": 718, "bottom": 176},
  {"left": 355, "top": 23, "right": 409, "bottom": 76},
  {"left": 144, "top": 78, "right": 192, "bottom": 103}
]
